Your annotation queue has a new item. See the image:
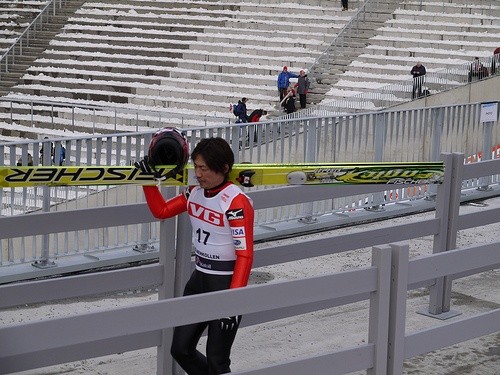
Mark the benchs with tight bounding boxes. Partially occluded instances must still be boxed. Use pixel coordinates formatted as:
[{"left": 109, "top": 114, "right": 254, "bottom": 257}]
[{"left": 0, "top": 0, "right": 500, "bottom": 217}]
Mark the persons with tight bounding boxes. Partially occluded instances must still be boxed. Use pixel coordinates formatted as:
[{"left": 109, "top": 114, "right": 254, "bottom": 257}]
[
  {"left": 17, "top": 136, "right": 67, "bottom": 167},
  {"left": 278, "top": 66, "right": 312, "bottom": 115},
  {"left": 410, "top": 61, "right": 426, "bottom": 100},
  {"left": 230, "top": 97, "right": 267, "bottom": 143},
  {"left": 466, "top": 47, "right": 500, "bottom": 82},
  {"left": 136, "top": 136, "right": 254, "bottom": 375}
]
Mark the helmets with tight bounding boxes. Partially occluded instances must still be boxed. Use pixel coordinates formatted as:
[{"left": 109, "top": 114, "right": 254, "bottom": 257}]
[{"left": 148, "top": 127, "right": 189, "bottom": 182}]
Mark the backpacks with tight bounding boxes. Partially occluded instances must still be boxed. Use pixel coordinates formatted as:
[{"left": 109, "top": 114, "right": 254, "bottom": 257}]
[{"left": 233, "top": 105, "right": 241, "bottom": 116}]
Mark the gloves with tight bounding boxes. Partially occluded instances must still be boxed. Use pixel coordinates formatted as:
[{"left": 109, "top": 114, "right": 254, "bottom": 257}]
[
  {"left": 217, "top": 317, "right": 236, "bottom": 331},
  {"left": 134, "top": 155, "right": 162, "bottom": 179}
]
